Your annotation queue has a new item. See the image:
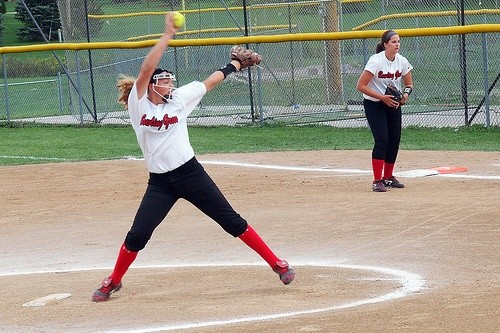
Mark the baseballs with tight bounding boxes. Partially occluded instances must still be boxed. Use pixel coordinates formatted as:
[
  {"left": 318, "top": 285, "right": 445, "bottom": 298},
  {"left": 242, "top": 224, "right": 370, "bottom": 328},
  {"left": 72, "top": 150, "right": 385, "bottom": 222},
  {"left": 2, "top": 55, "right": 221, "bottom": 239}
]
[{"left": 172, "top": 12, "right": 185, "bottom": 28}]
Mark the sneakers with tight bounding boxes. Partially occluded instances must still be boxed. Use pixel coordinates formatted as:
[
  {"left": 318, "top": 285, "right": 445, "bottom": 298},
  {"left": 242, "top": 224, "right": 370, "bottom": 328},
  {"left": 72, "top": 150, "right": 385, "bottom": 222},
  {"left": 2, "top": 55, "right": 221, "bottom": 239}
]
[
  {"left": 372, "top": 180, "right": 386, "bottom": 191},
  {"left": 382, "top": 176, "right": 405, "bottom": 188},
  {"left": 272, "top": 260, "right": 295, "bottom": 285},
  {"left": 91, "top": 277, "right": 121, "bottom": 302}
]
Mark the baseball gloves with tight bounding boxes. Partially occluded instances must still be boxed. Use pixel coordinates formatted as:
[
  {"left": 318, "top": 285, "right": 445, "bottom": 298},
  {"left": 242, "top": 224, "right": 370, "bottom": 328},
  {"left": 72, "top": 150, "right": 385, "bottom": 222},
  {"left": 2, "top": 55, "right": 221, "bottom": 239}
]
[
  {"left": 384, "top": 84, "right": 405, "bottom": 105},
  {"left": 229, "top": 44, "right": 262, "bottom": 69}
]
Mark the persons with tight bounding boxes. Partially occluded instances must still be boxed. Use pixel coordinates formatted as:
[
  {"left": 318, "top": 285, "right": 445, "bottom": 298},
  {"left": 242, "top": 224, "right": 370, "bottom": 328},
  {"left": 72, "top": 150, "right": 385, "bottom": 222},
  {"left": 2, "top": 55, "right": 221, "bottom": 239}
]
[
  {"left": 356, "top": 30, "right": 413, "bottom": 193},
  {"left": 92, "top": 12, "right": 294, "bottom": 302}
]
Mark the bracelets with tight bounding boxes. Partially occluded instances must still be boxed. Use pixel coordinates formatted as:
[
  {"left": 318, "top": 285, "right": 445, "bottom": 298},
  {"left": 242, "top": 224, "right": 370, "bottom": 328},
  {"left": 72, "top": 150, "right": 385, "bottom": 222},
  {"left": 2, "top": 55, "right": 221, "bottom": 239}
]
[
  {"left": 404, "top": 86, "right": 413, "bottom": 95},
  {"left": 217, "top": 63, "right": 237, "bottom": 80}
]
